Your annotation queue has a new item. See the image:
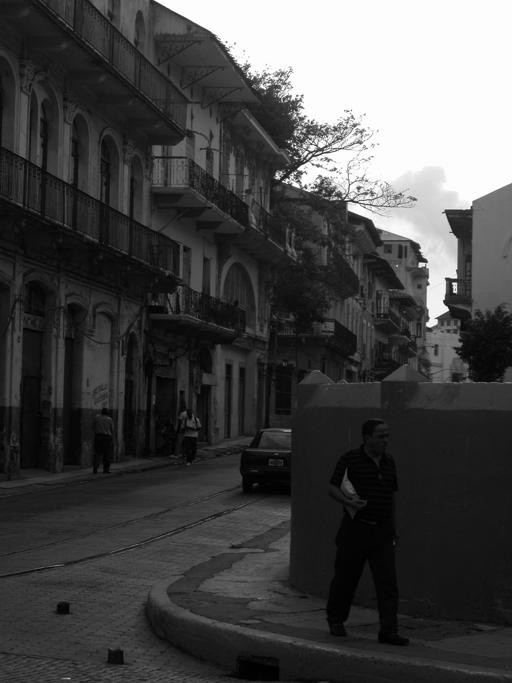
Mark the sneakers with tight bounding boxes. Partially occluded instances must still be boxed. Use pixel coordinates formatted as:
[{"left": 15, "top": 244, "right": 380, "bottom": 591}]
[
  {"left": 326, "top": 617, "right": 348, "bottom": 637},
  {"left": 378, "top": 631, "right": 409, "bottom": 646}
]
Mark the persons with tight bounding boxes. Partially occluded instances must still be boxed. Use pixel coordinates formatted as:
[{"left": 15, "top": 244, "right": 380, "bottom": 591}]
[
  {"left": 180, "top": 408, "right": 203, "bottom": 467},
  {"left": 325, "top": 418, "right": 411, "bottom": 647},
  {"left": 90, "top": 407, "right": 115, "bottom": 474},
  {"left": 167, "top": 405, "right": 187, "bottom": 458}
]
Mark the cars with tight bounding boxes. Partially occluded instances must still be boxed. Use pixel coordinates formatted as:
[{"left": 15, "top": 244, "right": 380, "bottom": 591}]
[{"left": 239, "top": 428, "right": 292, "bottom": 496}]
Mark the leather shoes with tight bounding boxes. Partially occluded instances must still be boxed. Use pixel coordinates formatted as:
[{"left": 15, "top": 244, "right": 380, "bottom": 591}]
[{"left": 92, "top": 459, "right": 192, "bottom": 474}]
